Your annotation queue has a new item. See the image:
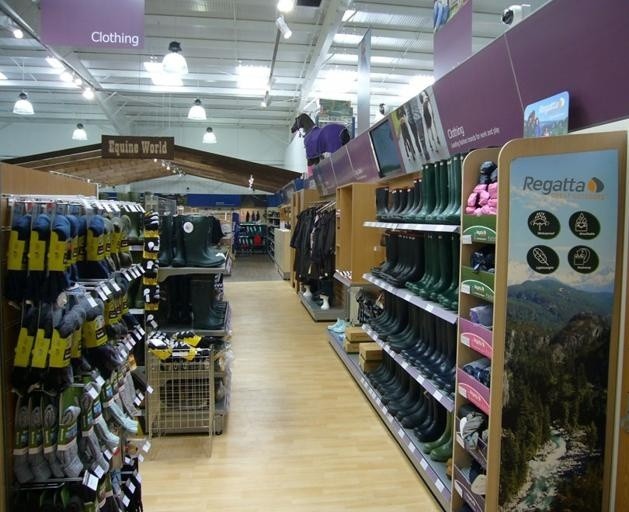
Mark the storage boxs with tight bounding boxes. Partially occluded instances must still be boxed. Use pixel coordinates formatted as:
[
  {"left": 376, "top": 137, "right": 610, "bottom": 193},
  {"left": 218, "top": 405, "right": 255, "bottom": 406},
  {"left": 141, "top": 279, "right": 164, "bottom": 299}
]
[
  {"left": 358, "top": 342, "right": 384, "bottom": 360},
  {"left": 359, "top": 355, "right": 382, "bottom": 375},
  {"left": 346, "top": 326, "right": 375, "bottom": 342},
  {"left": 344, "top": 338, "right": 365, "bottom": 353}
]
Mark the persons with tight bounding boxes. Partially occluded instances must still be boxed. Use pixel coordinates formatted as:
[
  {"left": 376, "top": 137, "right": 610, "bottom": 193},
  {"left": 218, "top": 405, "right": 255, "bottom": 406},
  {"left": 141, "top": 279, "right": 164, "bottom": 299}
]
[
  {"left": 394, "top": 91, "right": 443, "bottom": 161},
  {"left": 524, "top": 111, "right": 571, "bottom": 137}
]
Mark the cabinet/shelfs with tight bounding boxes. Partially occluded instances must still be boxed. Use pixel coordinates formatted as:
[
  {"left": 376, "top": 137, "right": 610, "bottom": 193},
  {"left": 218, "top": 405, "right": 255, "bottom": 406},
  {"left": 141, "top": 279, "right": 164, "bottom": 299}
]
[
  {"left": 145, "top": 242, "right": 233, "bottom": 434},
  {"left": 289, "top": 189, "right": 322, "bottom": 294},
  {"left": 335, "top": 181, "right": 382, "bottom": 287},
  {"left": 264, "top": 206, "right": 283, "bottom": 259},
  {"left": 450, "top": 127, "right": 628, "bottom": 512},
  {"left": 361, "top": 163, "right": 454, "bottom": 510}
]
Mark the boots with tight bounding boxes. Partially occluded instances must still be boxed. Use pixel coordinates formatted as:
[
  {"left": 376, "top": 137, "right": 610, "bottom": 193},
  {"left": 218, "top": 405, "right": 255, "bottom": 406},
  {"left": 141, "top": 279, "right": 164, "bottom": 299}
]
[
  {"left": 376, "top": 153, "right": 461, "bottom": 221},
  {"left": 381, "top": 372, "right": 454, "bottom": 462},
  {"left": 367, "top": 294, "right": 456, "bottom": 393},
  {"left": 188, "top": 279, "right": 227, "bottom": 330},
  {"left": 370, "top": 232, "right": 459, "bottom": 309},
  {"left": 159, "top": 216, "right": 225, "bottom": 267}
]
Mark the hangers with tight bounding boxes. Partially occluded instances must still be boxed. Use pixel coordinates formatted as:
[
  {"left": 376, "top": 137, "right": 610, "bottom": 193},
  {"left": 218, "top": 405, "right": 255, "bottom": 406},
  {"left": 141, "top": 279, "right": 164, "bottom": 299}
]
[{"left": 308, "top": 198, "right": 337, "bottom": 214}]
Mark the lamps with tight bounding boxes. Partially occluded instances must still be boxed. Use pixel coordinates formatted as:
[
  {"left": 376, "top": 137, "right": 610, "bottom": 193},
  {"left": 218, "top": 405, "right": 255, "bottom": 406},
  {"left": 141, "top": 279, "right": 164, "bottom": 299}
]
[
  {"left": 160, "top": 42, "right": 188, "bottom": 79},
  {"left": 72, "top": 122, "right": 88, "bottom": 141},
  {"left": 12, "top": 92, "right": 35, "bottom": 115},
  {"left": 13, "top": 27, "right": 22, "bottom": 39},
  {"left": 47, "top": 56, "right": 95, "bottom": 100},
  {"left": 231, "top": 2, "right": 307, "bottom": 145},
  {"left": 200, "top": 127, "right": 217, "bottom": 145},
  {"left": 188, "top": 99, "right": 208, "bottom": 123}
]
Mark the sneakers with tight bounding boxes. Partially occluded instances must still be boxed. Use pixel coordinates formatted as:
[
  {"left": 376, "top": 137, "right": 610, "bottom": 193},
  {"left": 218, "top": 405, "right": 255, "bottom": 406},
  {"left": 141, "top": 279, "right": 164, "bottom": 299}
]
[
  {"left": 334, "top": 322, "right": 352, "bottom": 333},
  {"left": 327, "top": 318, "right": 345, "bottom": 331}
]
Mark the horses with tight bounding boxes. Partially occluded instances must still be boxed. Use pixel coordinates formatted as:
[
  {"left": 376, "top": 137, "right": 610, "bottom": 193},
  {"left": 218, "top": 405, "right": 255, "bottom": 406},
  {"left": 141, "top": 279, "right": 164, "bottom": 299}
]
[{"left": 290, "top": 111, "right": 350, "bottom": 166}]
